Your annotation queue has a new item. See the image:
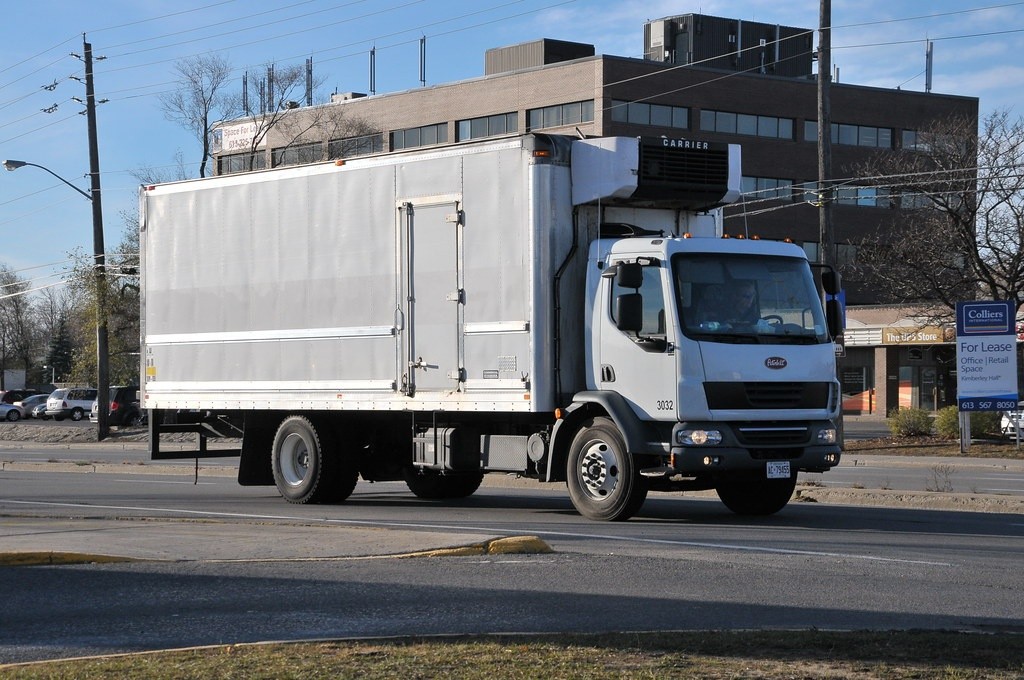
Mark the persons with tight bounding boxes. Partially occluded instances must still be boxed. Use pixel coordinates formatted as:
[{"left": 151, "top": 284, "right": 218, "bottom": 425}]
[{"left": 718, "top": 279, "right": 761, "bottom": 324}]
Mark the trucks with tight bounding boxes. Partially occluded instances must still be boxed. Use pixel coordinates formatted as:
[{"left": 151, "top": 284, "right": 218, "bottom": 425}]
[{"left": 138, "top": 127, "right": 844, "bottom": 521}]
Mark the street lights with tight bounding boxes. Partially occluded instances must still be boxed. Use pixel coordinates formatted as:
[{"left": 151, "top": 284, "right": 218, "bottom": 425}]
[{"left": 2, "top": 158, "right": 110, "bottom": 441}]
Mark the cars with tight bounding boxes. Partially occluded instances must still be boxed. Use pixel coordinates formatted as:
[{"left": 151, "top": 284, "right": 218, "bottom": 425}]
[
  {"left": 13, "top": 394, "right": 51, "bottom": 419},
  {"left": 0, "top": 388, "right": 39, "bottom": 422},
  {"left": 32, "top": 403, "right": 53, "bottom": 421},
  {"left": 1000, "top": 400, "right": 1024, "bottom": 441}
]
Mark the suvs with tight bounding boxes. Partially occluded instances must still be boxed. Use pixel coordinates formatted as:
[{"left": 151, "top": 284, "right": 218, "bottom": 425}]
[
  {"left": 45, "top": 387, "right": 98, "bottom": 421},
  {"left": 88, "top": 385, "right": 164, "bottom": 427}
]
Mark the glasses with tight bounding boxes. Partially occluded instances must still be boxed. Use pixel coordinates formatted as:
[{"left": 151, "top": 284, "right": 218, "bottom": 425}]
[{"left": 742, "top": 294, "right": 753, "bottom": 298}]
